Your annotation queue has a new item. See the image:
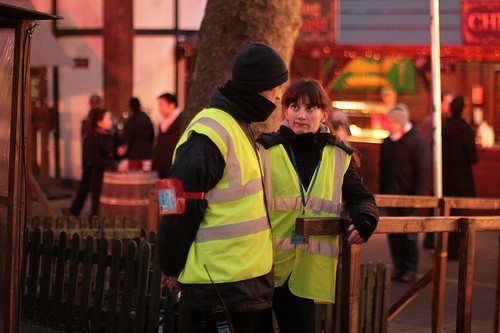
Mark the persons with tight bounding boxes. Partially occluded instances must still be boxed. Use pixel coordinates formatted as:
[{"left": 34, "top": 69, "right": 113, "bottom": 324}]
[
  {"left": 255, "top": 77, "right": 380, "bottom": 333},
  {"left": 60, "top": 94, "right": 184, "bottom": 223},
  {"left": 377, "top": 90, "right": 479, "bottom": 283},
  {"left": 155, "top": 41, "right": 289, "bottom": 333}
]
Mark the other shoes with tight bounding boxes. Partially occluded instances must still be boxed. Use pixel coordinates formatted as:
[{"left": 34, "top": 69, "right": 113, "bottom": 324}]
[
  {"left": 400, "top": 272, "right": 415, "bottom": 281},
  {"left": 391, "top": 271, "right": 400, "bottom": 279}
]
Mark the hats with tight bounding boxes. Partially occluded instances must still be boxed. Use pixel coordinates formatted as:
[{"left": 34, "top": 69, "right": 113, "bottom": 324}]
[
  {"left": 231, "top": 42, "right": 288, "bottom": 93},
  {"left": 388, "top": 104, "right": 407, "bottom": 125}
]
